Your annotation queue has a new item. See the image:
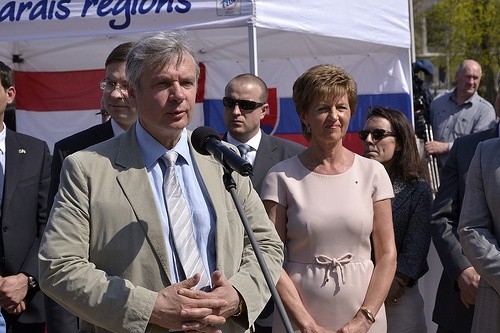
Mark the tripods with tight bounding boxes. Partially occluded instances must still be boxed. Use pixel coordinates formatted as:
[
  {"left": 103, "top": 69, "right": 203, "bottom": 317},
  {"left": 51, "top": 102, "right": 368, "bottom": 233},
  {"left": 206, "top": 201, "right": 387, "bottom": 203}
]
[{"left": 419, "top": 115, "right": 440, "bottom": 201}]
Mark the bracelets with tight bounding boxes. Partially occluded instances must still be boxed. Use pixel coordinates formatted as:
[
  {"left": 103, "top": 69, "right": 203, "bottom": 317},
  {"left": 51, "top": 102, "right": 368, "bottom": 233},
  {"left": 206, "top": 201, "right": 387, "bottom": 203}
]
[
  {"left": 360, "top": 306, "right": 375, "bottom": 323},
  {"left": 233, "top": 295, "right": 247, "bottom": 318},
  {"left": 396, "top": 276, "right": 406, "bottom": 288}
]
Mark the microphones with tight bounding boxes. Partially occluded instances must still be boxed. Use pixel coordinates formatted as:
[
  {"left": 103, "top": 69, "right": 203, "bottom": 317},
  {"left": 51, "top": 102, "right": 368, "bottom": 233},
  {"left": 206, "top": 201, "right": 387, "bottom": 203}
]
[{"left": 191, "top": 126, "right": 253, "bottom": 177}]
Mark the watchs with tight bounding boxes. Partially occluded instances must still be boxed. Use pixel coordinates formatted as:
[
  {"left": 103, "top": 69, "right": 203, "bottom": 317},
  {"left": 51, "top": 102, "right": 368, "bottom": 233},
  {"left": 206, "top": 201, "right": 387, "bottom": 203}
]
[{"left": 22, "top": 272, "right": 37, "bottom": 290}]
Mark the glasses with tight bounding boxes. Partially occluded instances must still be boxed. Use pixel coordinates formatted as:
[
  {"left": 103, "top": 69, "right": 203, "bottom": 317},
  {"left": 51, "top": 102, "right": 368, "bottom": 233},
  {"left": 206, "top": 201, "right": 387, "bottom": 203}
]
[
  {"left": 222, "top": 97, "right": 266, "bottom": 110},
  {"left": 358, "top": 128, "right": 398, "bottom": 141},
  {"left": 95, "top": 110, "right": 109, "bottom": 122},
  {"left": 99, "top": 79, "right": 129, "bottom": 93}
]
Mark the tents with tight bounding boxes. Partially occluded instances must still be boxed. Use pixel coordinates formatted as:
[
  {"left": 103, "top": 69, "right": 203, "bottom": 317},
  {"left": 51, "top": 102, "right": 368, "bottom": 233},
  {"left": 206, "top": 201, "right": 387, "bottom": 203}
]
[{"left": 1, "top": 0, "right": 415, "bottom": 159}]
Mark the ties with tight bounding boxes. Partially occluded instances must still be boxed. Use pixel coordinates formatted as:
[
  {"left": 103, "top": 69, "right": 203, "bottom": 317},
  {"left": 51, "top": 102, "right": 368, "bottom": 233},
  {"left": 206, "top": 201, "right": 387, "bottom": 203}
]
[
  {"left": 0, "top": 150, "right": 4, "bottom": 207},
  {"left": 162, "top": 150, "right": 212, "bottom": 293},
  {"left": 237, "top": 144, "right": 253, "bottom": 163}
]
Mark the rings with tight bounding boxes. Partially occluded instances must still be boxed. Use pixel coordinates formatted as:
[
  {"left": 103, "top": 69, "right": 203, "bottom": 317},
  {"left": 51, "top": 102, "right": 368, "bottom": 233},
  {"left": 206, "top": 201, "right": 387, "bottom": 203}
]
[{"left": 392, "top": 299, "right": 399, "bottom": 303}]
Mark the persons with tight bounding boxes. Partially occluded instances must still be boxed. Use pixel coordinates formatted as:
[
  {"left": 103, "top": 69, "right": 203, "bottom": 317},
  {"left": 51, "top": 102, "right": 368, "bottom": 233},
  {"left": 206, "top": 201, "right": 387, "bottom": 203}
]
[{"left": 0, "top": 32, "right": 500, "bottom": 333}]
